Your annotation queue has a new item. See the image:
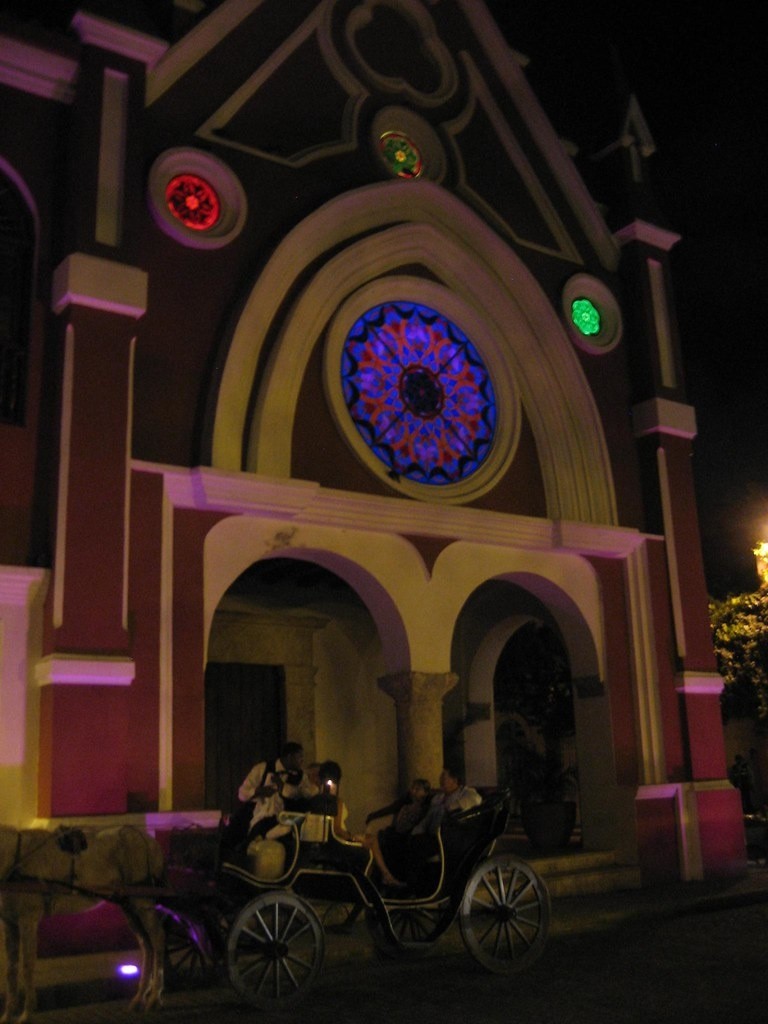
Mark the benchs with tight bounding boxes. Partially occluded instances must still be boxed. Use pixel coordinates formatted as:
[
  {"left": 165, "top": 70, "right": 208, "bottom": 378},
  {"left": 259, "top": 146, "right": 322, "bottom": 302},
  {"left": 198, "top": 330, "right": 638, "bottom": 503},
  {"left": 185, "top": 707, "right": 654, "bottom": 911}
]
[{"left": 246, "top": 786, "right": 510, "bottom": 875}]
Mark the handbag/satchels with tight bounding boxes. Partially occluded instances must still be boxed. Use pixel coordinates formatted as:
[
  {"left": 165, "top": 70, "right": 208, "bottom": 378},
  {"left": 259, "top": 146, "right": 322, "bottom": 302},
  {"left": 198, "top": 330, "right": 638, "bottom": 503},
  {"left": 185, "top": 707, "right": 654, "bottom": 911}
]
[{"left": 219, "top": 801, "right": 256, "bottom": 861}]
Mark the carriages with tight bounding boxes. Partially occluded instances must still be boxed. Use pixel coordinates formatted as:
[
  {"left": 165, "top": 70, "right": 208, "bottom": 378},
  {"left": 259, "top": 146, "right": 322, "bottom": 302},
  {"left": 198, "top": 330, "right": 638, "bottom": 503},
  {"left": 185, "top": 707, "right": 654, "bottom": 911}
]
[{"left": 0, "top": 778, "right": 552, "bottom": 1024}]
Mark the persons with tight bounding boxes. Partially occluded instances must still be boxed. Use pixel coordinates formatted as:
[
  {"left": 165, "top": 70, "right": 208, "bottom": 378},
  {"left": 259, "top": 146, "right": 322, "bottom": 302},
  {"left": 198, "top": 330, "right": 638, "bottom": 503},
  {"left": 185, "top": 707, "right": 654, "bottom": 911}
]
[{"left": 215, "top": 742, "right": 483, "bottom": 888}]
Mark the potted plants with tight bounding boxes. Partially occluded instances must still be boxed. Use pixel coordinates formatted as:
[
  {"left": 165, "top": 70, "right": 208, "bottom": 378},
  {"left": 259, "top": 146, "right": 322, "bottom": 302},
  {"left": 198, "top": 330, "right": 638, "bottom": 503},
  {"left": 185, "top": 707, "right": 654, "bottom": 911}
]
[{"left": 505, "top": 745, "right": 577, "bottom": 853}]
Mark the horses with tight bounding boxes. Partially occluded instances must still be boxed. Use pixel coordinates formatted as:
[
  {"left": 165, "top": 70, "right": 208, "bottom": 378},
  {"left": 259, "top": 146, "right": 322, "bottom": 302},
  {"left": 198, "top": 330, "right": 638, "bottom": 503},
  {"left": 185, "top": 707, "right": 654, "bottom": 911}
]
[{"left": 0, "top": 823, "right": 164, "bottom": 1024}]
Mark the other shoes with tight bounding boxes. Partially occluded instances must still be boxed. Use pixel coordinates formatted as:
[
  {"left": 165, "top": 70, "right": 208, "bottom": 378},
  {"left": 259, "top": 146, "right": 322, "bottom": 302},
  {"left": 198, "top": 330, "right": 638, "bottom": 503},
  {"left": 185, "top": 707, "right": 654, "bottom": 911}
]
[{"left": 381, "top": 881, "right": 406, "bottom": 889}]
